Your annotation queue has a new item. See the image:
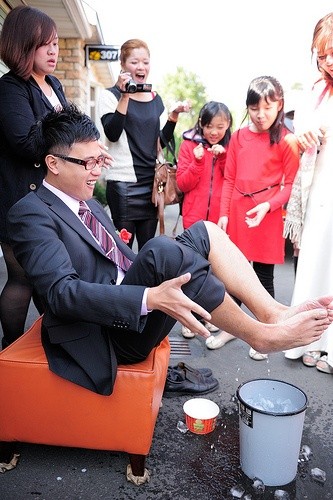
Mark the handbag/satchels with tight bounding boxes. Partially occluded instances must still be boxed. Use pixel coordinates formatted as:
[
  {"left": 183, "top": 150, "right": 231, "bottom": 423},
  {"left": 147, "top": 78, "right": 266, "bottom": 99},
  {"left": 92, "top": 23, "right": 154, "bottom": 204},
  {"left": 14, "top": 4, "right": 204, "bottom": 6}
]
[{"left": 152, "top": 136, "right": 183, "bottom": 206}]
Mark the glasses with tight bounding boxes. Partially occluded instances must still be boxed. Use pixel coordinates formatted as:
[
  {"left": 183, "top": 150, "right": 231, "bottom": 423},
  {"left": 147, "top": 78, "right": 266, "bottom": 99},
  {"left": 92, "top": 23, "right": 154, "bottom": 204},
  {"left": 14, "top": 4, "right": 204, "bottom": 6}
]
[{"left": 45, "top": 152, "right": 105, "bottom": 170}]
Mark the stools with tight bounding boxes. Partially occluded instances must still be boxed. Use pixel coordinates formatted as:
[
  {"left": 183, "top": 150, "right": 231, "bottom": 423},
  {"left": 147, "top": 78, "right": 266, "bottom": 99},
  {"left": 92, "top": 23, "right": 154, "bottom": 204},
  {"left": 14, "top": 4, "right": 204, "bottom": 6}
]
[{"left": 0, "top": 313, "right": 170, "bottom": 485}]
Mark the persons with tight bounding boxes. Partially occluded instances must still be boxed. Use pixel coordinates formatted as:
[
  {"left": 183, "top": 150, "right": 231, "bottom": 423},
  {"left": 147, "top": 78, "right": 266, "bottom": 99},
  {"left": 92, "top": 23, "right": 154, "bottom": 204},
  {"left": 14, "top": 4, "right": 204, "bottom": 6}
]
[
  {"left": 96, "top": 39, "right": 191, "bottom": 254},
  {"left": 0, "top": 6, "right": 116, "bottom": 350},
  {"left": 283, "top": 12, "right": 332, "bottom": 374},
  {"left": 205, "top": 74, "right": 300, "bottom": 360},
  {"left": 6, "top": 102, "right": 333, "bottom": 396},
  {"left": 175, "top": 102, "right": 233, "bottom": 339}
]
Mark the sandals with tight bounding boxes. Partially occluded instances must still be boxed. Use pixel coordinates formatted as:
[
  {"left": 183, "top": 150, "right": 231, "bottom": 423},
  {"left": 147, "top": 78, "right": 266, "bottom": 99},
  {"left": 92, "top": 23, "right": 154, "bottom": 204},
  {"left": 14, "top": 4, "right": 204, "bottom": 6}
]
[{"left": 303, "top": 352, "right": 333, "bottom": 373}]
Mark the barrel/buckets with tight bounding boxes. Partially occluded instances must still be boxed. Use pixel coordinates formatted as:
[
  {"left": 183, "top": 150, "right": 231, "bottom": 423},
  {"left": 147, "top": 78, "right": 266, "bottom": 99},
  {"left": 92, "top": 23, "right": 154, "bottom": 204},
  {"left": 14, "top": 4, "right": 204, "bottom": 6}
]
[{"left": 236, "top": 378, "right": 309, "bottom": 486}]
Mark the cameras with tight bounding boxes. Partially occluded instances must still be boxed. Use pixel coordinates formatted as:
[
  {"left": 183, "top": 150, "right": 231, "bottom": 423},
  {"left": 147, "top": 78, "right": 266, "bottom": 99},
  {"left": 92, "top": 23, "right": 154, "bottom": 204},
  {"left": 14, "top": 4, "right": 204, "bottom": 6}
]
[{"left": 122, "top": 71, "right": 152, "bottom": 93}]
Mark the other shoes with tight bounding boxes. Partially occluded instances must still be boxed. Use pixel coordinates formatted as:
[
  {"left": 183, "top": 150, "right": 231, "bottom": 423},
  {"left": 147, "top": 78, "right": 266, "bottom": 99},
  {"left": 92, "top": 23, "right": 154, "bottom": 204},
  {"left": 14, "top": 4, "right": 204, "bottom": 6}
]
[
  {"left": 181, "top": 321, "right": 221, "bottom": 338},
  {"left": 248, "top": 348, "right": 268, "bottom": 361},
  {"left": 206, "top": 330, "right": 236, "bottom": 350}
]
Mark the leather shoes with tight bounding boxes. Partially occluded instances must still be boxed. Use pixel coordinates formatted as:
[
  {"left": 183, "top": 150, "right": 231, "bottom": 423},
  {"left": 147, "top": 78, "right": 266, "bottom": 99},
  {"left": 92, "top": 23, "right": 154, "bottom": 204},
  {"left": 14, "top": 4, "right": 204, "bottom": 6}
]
[{"left": 162, "top": 363, "right": 218, "bottom": 398}]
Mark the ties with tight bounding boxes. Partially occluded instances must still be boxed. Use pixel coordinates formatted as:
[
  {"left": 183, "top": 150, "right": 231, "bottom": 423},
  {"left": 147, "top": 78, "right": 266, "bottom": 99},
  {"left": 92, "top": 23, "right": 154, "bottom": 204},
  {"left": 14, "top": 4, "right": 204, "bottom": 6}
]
[{"left": 78, "top": 201, "right": 133, "bottom": 273}]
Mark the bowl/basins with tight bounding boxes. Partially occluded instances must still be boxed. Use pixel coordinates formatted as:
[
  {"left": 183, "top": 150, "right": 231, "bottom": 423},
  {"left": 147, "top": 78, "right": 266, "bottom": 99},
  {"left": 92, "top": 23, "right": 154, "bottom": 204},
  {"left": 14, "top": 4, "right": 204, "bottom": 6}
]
[{"left": 183, "top": 398, "right": 220, "bottom": 434}]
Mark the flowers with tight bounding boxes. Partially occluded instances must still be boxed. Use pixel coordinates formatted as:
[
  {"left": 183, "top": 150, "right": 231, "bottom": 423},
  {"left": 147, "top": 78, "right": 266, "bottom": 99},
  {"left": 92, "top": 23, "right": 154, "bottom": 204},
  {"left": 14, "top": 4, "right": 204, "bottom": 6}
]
[{"left": 115, "top": 228, "right": 132, "bottom": 245}]
